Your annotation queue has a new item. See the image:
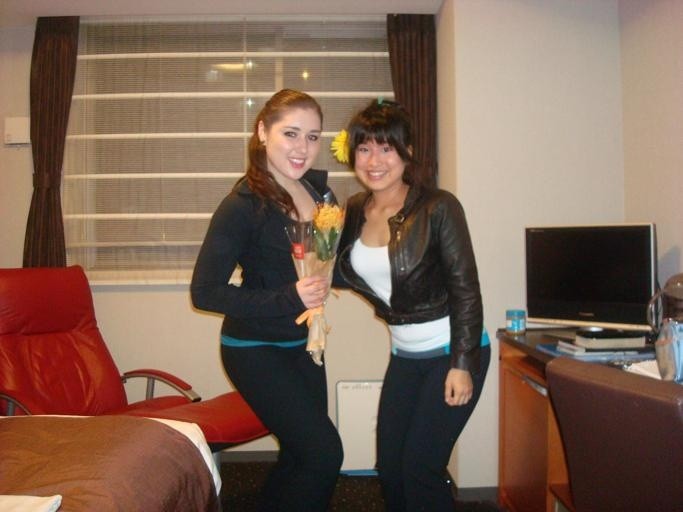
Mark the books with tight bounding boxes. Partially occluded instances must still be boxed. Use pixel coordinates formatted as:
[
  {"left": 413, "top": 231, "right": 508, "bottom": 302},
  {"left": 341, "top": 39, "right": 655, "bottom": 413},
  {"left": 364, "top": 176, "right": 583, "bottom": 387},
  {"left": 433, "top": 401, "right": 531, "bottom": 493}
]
[
  {"left": 569, "top": 332, "right": 646, "bottom": 349},
  {"left": 556, "top": 337, "right": 592, "bottom": 353},
  {"left": 534, "top": 340, "right": 625, "bottom": 361}
]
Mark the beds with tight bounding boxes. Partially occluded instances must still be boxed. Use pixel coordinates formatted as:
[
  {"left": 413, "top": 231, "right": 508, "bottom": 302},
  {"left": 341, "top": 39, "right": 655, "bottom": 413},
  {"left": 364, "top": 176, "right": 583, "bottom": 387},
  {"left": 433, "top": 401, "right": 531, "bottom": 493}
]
[{"left": 0, "top": 413, "right": 211, "bottom": 512}]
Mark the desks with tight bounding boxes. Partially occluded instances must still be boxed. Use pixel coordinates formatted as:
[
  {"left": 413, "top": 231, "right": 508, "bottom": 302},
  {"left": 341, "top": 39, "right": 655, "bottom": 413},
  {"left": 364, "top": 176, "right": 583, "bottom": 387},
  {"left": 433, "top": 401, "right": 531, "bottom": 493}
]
[{"left": 495, "top": 324, "right": 683, "bottom": 511}]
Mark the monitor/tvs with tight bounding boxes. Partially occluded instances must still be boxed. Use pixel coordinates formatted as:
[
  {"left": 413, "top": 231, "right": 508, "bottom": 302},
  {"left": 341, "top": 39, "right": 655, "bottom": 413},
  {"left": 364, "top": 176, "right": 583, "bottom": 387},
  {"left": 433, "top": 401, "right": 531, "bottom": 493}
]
[{"left": 524, "top": 223, "right": 658, "bottom": 341}]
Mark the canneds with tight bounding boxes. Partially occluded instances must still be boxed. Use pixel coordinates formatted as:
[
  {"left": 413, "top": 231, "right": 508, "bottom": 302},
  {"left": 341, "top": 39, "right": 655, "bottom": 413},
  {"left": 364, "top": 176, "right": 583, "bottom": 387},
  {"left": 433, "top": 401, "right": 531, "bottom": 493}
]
[{"left": 506, "top": 309, "right": 526, "bottom": 337}]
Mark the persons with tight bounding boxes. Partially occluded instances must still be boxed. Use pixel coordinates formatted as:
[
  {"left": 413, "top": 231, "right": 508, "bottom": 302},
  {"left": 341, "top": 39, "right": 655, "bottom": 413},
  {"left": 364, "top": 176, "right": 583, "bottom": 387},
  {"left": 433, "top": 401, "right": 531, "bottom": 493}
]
[
  {"left": 189, "top": 87, "right": 347, "bottom": 512},
  {"left": 326, "top": 98, "right": 495, "bottom": 512}
]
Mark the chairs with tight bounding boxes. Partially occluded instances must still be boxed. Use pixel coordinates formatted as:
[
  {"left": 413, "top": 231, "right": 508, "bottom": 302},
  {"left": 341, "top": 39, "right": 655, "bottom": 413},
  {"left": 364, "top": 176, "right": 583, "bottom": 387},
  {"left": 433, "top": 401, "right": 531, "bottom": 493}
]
[
  {"left": 542, "top": 355, "right": 683, "bottom": 512},
  {"left": 0, "top": 264, "right": 273, "bottom": 454}
]
